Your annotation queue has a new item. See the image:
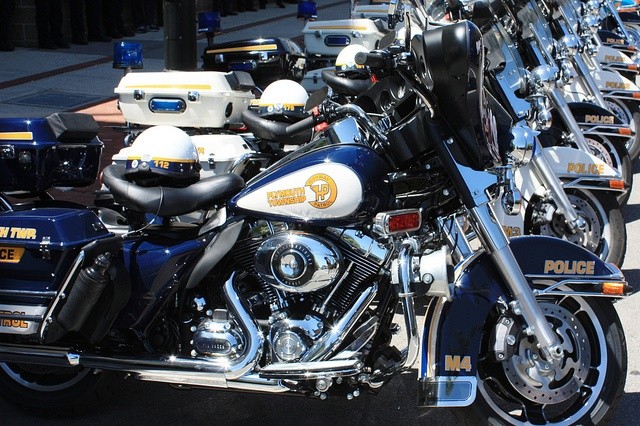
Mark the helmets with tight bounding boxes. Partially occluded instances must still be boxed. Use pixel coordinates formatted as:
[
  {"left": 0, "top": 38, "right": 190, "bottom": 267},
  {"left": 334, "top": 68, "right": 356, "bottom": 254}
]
[
  {"left": 336, "top": 44, "right": 369, "bottom": 79},
  {"left": 259, "top": 79, "right": 309, "bottom": 119},
  {"left": 125, "top": 125, "right": 199, "bottom": 186}
]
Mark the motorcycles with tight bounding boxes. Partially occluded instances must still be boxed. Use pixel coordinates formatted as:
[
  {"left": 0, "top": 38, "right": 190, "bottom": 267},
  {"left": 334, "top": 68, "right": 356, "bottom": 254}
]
[
  {"left": 0, "top": 13, "right": 636, "bottom": 426},
  {"left": 0, "top": 0, "right": 640, "bottom": 308}
]
